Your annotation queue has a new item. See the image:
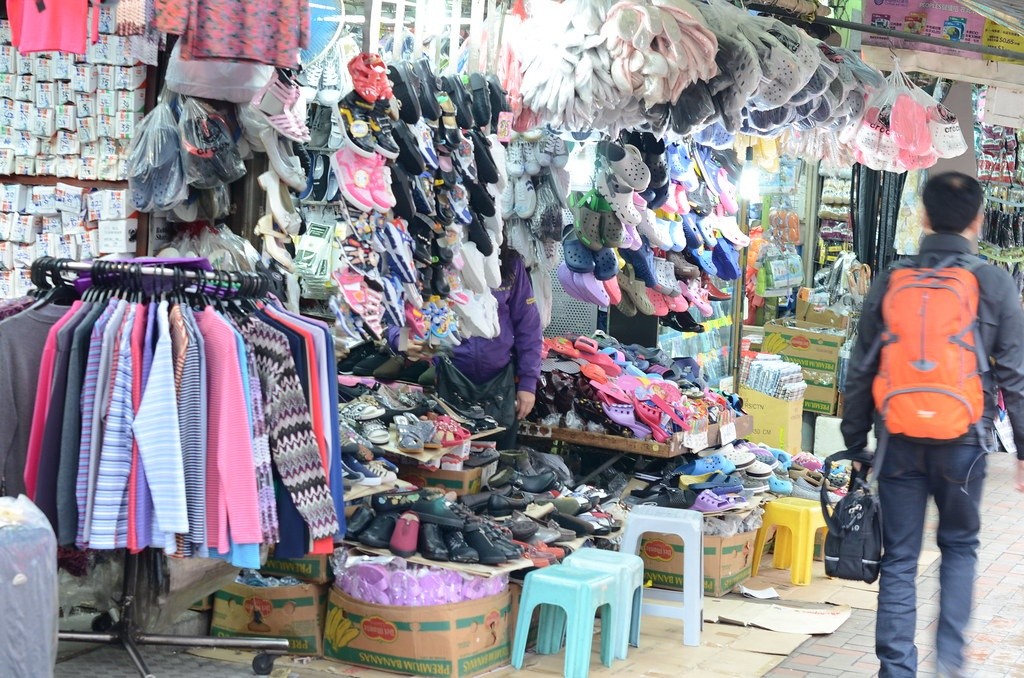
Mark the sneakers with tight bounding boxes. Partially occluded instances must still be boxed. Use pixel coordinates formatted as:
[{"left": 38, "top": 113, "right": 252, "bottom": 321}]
[{"left": 332, "top": 94, "right": 477, "bottom": 328}]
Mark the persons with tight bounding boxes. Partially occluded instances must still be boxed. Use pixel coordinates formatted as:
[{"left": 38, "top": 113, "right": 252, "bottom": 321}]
[
  {"left": 384, "top": 242, "right": 544, "bottom": 451},
  {"left": 836, "top": 169, "right": 1024, "bottom": 678}
]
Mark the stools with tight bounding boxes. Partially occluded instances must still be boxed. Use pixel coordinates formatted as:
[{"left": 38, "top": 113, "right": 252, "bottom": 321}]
[
  {"left": 510, "top": 564, "right": 618, "bottom": 678},
  {"left": 751, "top": 497, "right": 836, "bottom": 586},
  {"left": 620, "top": 505, "right": 705, "bottom": 647},
  {"left": 560, "top": 547, "right": 644, "bottom": 661}
]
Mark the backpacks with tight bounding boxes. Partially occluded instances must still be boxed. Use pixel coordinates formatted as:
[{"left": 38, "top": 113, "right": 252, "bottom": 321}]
[{"left": 870, "top": 253, "right": 990, "bottom": 439}]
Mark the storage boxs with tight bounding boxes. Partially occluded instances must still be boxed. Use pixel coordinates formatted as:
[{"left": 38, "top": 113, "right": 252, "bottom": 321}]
[
  {"left": 761, "top": 318, "right": 846, "bottom": 375},
  {"left": 398, "top": 464, "right": 482, "bottom": 502},
  {"left": 14, "top": 268, "right": 37, "bottom": 298},
  {"left": 796, "top": 286, "right": 849, "bottom": 329},
  {"left": 813, "top": 417, "right": 877, "bottom": 465},
  {"left": 322, "top": 585, "right": 514, "bottom": 678},
  {"left": 210, "top": 581, "right": 328, "bottom": 660},
  {"left": 0, "top": 0, "right": 148, "bottom": 269},
  {"left": 258, "top": 548, "right": 335, "bottom": 585},
  {"left": 0, "top": 271, "right": 14, "bottom": 299},
  {"left": 738, "top": 384, "right": 804, "bottom": 455},
  {"left": 801, "top": 368, "right": 839, "bottom": 415},
  {"left": 639, "top": 530, "right": 757, "bottom": 598}
]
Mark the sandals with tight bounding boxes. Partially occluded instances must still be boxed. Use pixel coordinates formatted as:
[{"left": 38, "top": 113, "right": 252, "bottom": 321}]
[
  {"left": 359, "top": 288, "right": 384, "bottom": 340},
  {"left": 340, "top": 377, "right": 497, "bottom": 452},
  {"left": 332, "top": 265, "right": 364, "bottom": 313},
  {"left": 248, "top": 71, "right": 304, "bottom": 143},
  {"left": 348, "top": 55, "right": 379, "bottom": 103},
  {"left": 371, "top": 54, "right": 392, "bottom": 101},
  {"left": 291, "top": 77, "right": 311, "bottom": 142}
]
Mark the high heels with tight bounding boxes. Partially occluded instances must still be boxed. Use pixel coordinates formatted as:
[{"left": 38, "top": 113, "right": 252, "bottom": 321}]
[
  {"left": 259, "top": 171, "right": 290, "bottom": 235},
  {"left": 261, "top": 236, "right": 296, "bottom": 274},
  {"left": 280, "top": 182, "right": 303, "bottom": 233},
  {"left": 253, "top": 215, "right": 291, "bottom": 268}
]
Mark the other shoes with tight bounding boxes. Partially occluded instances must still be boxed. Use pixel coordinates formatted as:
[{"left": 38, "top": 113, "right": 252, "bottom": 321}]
[
  {"left": 341, "top": 450, "right": 619, "bottom": 567},
  {"left": 302, "top": 52, "right": 498, "bottom": 376},
  {"left": 501, "top": 125, "right": 572, "bottom": 267},
  {"left": 257, "top": 128, "right": 300, "bottom": 188},
  {"left": 280, "top": 142, "right": 308, "bottom": 192}
]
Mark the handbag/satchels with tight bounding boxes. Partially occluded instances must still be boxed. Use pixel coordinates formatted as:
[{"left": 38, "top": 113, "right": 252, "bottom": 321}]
[
  {"left": 435, "top": 355, "right": 514, "bottom": 433},
  {"left": 165, "top": 36, "right": 273, "bottom": 103},
  {"left": 167, "top": 189, "right": 230, "bottom": 223},
  {"left": 825, "top": 464, "right": 884, "bottom": 583},
  {"left": 530, "top": 269, "right": 551, "bottom": 329},
  {"left": 156, "top": 226, "right": 259, "bottom": 272},
  {"left": 127, "top": 84, "right": 187, "bottom": 212},
  {"left": 480, "top": 0, "right": 966, "bottom": 176},
  {"left": 179, "top": 96, "right": 245, "bottom": 189}
]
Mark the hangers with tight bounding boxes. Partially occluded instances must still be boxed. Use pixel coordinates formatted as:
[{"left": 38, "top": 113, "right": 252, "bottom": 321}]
[{"left": 25, "top": 255, "right": 276, "bottom": 318}]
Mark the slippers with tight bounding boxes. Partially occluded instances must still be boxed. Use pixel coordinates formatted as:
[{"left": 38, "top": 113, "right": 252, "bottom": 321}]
[
  {"left": 515, "top": 329, "right": 854, "bottom": 536},
  {"left": 500, "top": 0, "right": 969, "bottom": 315},
  {"left": 403, "top": 300, "right": 457, "bottom": 341},
  {"left": 124, "top": 112, "right": 246, "bottom": 225},
  {"left": 329, "top": 548, "right": 501, "bottom": 607}
]
